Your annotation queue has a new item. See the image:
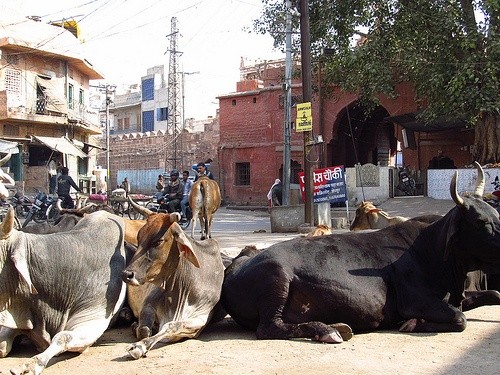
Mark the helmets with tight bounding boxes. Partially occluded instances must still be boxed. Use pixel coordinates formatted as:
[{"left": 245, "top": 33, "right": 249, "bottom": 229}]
[{"left": 170, "top": 169, "right": 180, "bottom": 176}]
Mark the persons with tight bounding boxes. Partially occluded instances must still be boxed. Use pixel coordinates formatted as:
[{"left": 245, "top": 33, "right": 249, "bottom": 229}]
[
  {"left": 267, "top": 179, "right": 280, "bottom": 207},
  {"left": 123, "top": 178, "right": 128, "bottom": 196},
  {"left": 191, "top": 162, "right": 213, "bottom": 182},
  {"left": 157, "top": 169, "right": 192, "bottom": 222},
  {"left": 58, "top": 167, "right": 81, "bottom": 210}
]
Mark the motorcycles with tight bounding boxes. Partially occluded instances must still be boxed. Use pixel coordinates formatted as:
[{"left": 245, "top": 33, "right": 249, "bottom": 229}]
[
  {"left": 22, "top": 187, "right": 55, "bottom": 228},
  {"left": 144, "top": 193, "right": 192, "bottom": 230},
  {"left": 397, "top": 166, "right": 416, "bottom": 195}
]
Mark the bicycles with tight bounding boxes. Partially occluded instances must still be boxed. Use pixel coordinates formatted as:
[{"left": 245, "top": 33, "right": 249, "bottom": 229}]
[{"left": 0, "top": 187, "right": 140, "bottom": 224}]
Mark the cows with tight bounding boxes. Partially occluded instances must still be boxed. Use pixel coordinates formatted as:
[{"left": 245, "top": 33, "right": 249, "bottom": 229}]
[
  {"left": 0, "top": 153, "right": 500, "bottom": 375},
  {"left": 187, "top": 167, "right": 222, "bottom": 241}
]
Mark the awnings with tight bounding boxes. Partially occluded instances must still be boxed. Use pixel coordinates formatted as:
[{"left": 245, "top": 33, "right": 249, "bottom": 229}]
[{"left": 32, "top": 136, "right": 88, "bottom": 158}]
